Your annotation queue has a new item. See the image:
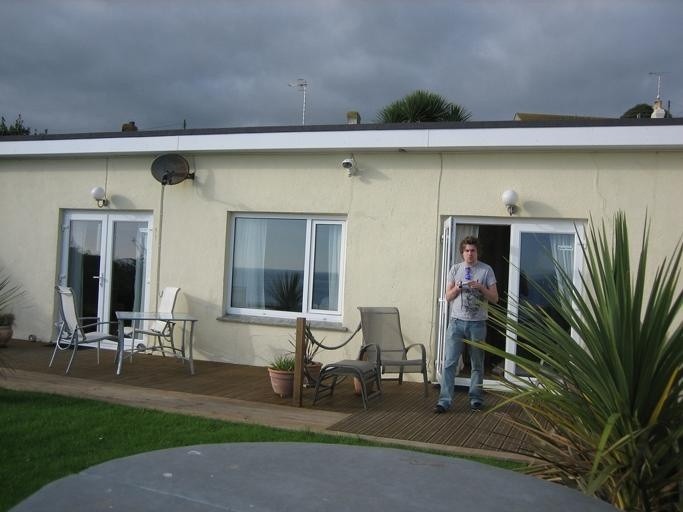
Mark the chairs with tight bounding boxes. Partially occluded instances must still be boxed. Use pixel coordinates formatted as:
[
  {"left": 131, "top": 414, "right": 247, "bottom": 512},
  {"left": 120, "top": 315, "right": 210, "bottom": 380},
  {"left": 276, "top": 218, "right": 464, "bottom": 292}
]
[
  {"left": 357, "top": 306, "right": 430, "bottom": 399},
  {"left": 48, "top": 285, "right": 120, "bottom": 376},
  {"left": 114, "top": 287, "right": 180, "bottom": 364}
]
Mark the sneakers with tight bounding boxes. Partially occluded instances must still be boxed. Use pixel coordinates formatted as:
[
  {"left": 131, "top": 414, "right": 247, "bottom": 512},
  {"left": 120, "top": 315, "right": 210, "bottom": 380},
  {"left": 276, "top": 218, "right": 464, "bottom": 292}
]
[
  {"left": 433, "top": 404, "right": 444, "bottom": 413},
  {"left": 471, "top": 402, "right": 482, "bottom": 412}
]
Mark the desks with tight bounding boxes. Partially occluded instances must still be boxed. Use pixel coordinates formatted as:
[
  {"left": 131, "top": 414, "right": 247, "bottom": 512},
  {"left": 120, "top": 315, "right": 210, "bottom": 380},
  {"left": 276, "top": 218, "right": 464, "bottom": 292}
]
[{"left": 115, "top": 312, "right": 199, "bottom": 376}]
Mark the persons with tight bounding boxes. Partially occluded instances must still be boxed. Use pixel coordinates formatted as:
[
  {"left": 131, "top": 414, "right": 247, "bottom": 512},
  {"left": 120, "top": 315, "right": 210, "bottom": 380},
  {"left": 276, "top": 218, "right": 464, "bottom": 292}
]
[{"left": 433, "top": 237, "right": 499, "bottom": 414}]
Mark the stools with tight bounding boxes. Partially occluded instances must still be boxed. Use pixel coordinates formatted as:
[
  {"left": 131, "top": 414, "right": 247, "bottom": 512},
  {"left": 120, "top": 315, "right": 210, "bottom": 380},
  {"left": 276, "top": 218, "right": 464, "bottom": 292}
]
[{"left": 312, "top": 359, "right": 383, "bottom": 412}]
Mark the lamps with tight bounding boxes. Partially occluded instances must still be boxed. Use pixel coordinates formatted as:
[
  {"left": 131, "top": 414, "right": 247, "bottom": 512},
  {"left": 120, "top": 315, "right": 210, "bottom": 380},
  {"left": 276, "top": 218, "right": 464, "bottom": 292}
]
[
  {"left": 91, "top": 185, "right": 109, "bottom": 209},
  {"left": 501, "top": 189, "right": 518, "bottom": 217}
]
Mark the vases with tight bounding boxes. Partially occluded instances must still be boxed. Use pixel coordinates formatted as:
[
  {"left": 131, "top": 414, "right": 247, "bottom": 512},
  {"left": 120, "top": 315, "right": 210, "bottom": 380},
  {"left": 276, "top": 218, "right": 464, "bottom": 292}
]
[
  {"left": 287, "top": 322, "right": 323, "bottom": 387},
  {"left": 458, "top": 208, "right": 683, "bottom": 512},
  {"left": 267, "top": 355, "right": 295, "bottom": 398}
]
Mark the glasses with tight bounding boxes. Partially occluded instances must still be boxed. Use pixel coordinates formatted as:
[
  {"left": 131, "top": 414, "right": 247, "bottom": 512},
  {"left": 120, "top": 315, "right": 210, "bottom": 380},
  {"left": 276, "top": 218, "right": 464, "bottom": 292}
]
[{"left": 465, "top": 267, "right": 470, "bottom": 280}]
